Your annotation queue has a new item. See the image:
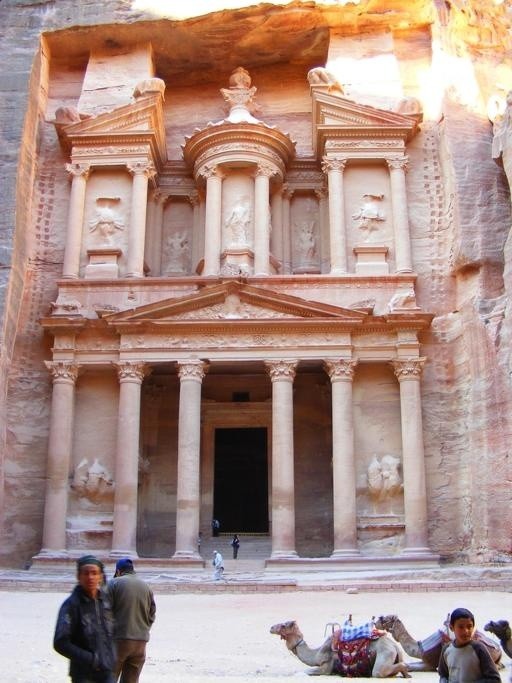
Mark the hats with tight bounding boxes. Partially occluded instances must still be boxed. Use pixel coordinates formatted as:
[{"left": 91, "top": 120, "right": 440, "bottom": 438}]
[{"left": 113, "top": 558, "right": 133, "bottom": 577}]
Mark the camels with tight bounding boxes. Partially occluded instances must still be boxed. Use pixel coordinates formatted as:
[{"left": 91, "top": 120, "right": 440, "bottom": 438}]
[
  {"left": 270, "top": 619, "right": 413, "bottom": 679},
  {"left": 376, "top": 614, "right": 512, "bottom": 673}
]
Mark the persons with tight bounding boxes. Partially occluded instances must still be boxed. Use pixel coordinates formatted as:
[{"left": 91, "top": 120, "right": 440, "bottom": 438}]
[
  {"left": 164, "top": 229, "right": 190, "bottom": 257},
  {"left": 211, "top": 518, "right": 221, "bottom": 537},
  {"left": 212, "top": 550, "right": 225, "bottom": 581},
  {"left": 230, "top": 534, "right": 241, "bottom": 560},
  {"left": 435, "top": 608, "right": 503, "bottom": 683},
  {"left": 198, "top": 532, "right": 203, "bottom": 552},
  {"left": 52, "top": 554, "right": 121, "bottom": 683},
  {"left": 104, "top": 558, "right": 157, "bottom": 683}
]
